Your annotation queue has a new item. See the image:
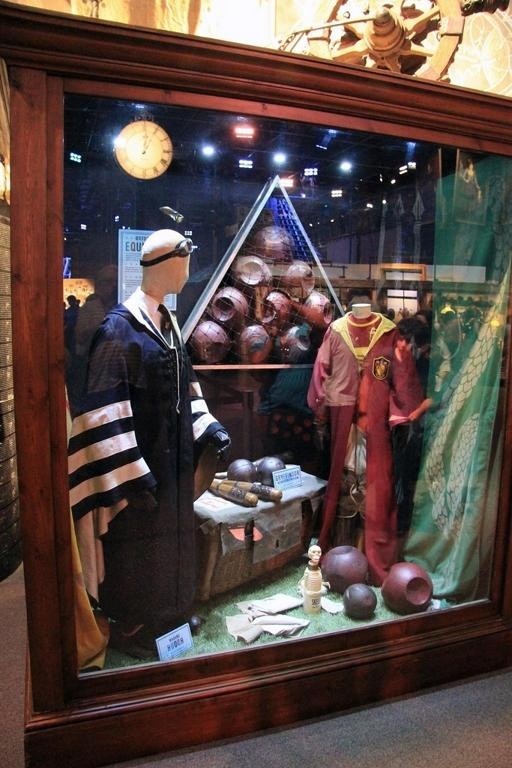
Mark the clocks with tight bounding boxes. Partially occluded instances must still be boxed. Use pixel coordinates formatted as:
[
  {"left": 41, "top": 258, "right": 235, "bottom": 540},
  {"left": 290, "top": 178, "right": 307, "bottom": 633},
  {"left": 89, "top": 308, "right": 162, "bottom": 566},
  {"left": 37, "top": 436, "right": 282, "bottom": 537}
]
[{"left": 114, "top": 122, "right": 174, "bottom": 181}]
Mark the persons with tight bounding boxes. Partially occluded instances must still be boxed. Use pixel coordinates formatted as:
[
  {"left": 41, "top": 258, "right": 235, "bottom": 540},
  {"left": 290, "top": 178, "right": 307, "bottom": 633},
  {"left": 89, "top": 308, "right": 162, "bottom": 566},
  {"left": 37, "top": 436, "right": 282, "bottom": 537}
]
[
  {"left": 307, "top": 301, "right": 431, "bottom": 582},
  {"left": 58, "top": 226, "right": 231, "bottom": 650},
  {"left": 65, "top": 280, "right": 510, "bottom": 553}
]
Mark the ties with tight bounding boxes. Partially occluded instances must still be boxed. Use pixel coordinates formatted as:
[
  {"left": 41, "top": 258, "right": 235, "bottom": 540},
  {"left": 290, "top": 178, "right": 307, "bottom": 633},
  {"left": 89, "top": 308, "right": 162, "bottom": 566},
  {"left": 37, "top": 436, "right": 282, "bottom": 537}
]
[{"left": 158, "top": 304, "right": 172, "bottom": 348}]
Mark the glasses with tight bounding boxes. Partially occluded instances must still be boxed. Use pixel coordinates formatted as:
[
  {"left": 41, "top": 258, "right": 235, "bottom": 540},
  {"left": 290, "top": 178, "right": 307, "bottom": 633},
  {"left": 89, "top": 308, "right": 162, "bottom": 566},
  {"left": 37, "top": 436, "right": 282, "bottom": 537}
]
[{"left": 139, "top": 237, "right": 194, "bottom": 266}]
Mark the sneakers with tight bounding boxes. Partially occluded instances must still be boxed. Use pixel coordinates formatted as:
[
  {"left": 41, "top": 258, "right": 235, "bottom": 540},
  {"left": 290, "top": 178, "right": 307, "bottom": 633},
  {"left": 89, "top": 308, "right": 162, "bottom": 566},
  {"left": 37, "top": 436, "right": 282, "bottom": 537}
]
[
  {"left": 115, "top": 621, "right": 157, "bottom": 661},
  {"left": 167, "top": 607, "right": 201, "bottom": 634}
]
